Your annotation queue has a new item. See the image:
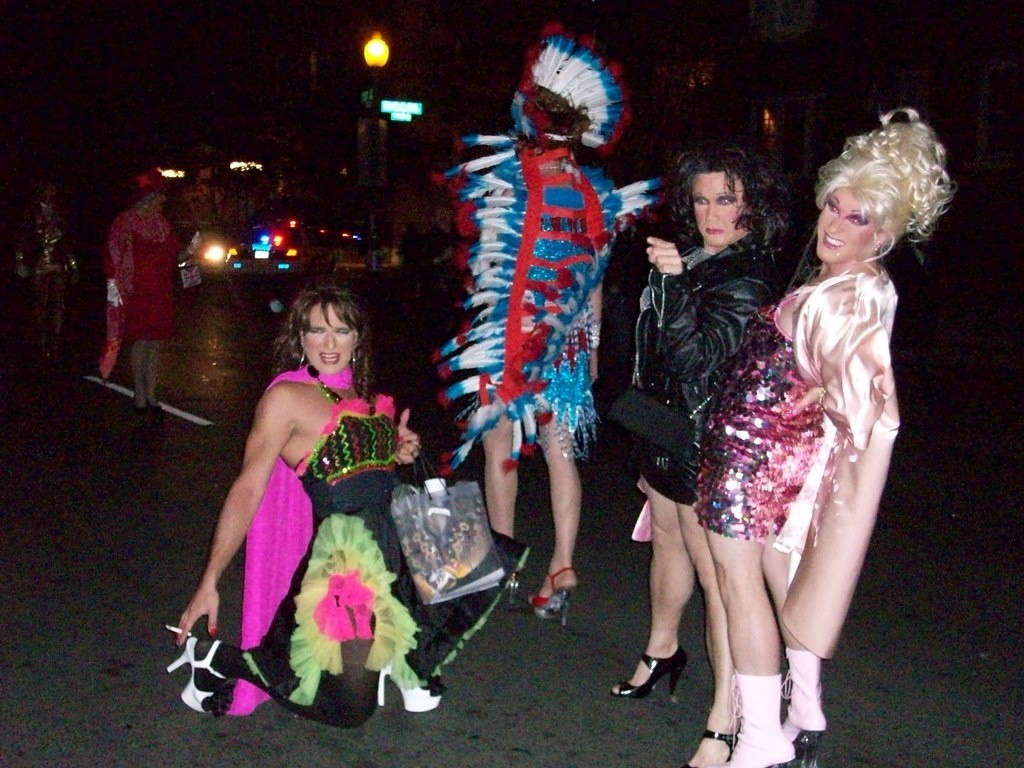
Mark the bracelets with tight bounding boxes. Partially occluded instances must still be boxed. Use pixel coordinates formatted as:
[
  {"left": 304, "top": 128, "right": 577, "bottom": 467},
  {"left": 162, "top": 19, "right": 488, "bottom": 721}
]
[
  {"left": 817, "top": 388, "right": 828, "bottom": 409},
  {"left": 590, "top": 321, "right": 601, "bottom": 349}
]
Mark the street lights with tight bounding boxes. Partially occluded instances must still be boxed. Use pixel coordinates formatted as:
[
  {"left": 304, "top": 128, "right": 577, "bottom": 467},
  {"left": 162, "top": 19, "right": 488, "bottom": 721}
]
[{"left": 362, "top": 31, "right": 390, "bottom": 242}]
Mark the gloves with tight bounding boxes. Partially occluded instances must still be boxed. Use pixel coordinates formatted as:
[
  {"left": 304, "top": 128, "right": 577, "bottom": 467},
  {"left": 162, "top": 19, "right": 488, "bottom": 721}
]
[
  {"left": 106, "top": 282, "right": 123, "bottom": 308},
  {"left": 188, "top": 232, "right": 202, "bottom": 254}
]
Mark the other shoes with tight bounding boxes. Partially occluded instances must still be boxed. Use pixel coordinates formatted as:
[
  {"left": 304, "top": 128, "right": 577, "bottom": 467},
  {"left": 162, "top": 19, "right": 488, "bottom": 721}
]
[{"left": 133, "top": 393, "right": 162, "bottom": 415}]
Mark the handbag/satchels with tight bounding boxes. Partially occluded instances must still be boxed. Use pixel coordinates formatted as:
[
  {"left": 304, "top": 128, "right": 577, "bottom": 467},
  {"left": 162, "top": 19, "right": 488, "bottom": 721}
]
[
  {"left": 606, "top": 384, "right": 696, "bottom": 462},
  {"left": 390, "top": 441, "right": 505, "bottom": 605},
  {"left": 180, "top": 265, "right": 202, "bottom": 289}
]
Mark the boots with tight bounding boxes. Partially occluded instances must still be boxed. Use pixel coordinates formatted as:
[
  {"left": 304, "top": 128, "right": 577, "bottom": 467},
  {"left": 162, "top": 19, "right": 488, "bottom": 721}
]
[
  {"left": 781, "top": 646, "right": 827, "bottom": 768},
  {"left": 706, "top": 669, "right": 796, "bottom": 768}
]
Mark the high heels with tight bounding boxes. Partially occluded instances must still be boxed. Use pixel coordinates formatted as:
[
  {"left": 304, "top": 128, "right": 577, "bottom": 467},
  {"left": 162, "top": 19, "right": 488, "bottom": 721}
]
[
  {"left": 680, "top": 729, "right": 741, "bottom": 768},
  {"left": 378, "top": 660, "right": 443, "bottom": 713},
  {"left": 532, "top": 567, "right": 573, "bottom": 626},
  {"left": 610, "top": 645, "right": 687, "bottom": 698},
  {"left": 501, "top": 571, "right": 523, "bottom": 606},
  {"left": 166, "top": 636, "right": 228, "bottom": 713}
]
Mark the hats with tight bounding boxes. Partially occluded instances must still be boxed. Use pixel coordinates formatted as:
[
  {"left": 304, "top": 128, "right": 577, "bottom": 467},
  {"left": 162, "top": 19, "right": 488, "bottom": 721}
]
[{"left": 125, "top": 165, "right": 177, "bottom": 204}]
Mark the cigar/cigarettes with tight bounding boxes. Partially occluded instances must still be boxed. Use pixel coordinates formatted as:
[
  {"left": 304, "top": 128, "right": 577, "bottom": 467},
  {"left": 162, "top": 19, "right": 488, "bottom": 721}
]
[{"left": 166, "top": 624, "right": 192, "bottom": 637}]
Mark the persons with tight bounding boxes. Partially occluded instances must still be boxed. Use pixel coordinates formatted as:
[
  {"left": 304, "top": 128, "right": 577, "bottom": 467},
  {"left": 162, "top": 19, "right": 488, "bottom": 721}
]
[
  {"left": 10, "top": 143, "right": 460, "bottom": 408},
  {"left": 610, "top": 136, "right": 789, "bottom": 768},
  {"left": 429, "top": 22, "right": 669, "bottom": 627},
  {"left": 167, "top": 282, "right": 529, "bottom": 728},
  {"left": 689, "top": 106, "right": 956, "bottom": 768}
]
